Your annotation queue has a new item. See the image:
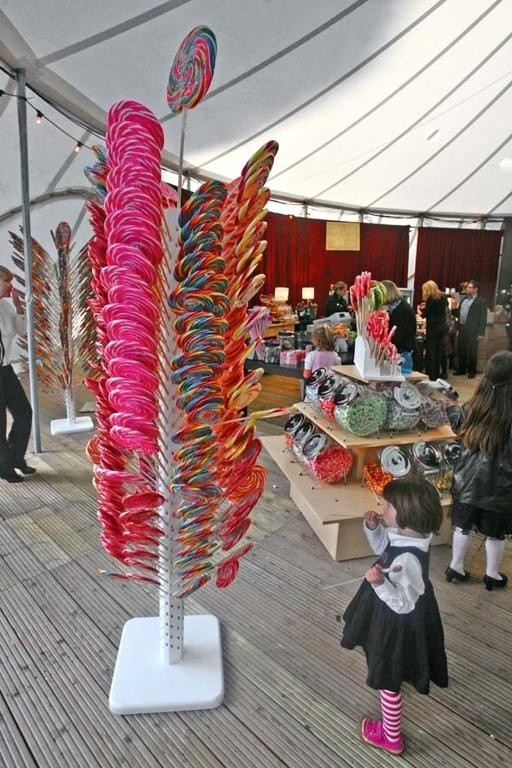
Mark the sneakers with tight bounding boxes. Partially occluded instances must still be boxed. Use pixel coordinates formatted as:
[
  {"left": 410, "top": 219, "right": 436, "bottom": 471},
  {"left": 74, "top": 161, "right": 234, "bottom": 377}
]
[
  {"left": 428, "top": 373, "right": 448, "bottom": 380},
  {"left": 1, "top": 468, "right": 23, "bottom": 484},
  {"left": 360, "top": 718, "right": 404, "bottom": 756},
  {"left": 16, "top": 461, "right": 37, "bottom": 473},
  {"left": 468, "top": 372, "right": 476, "bottom": 378},
  {"left": 453, "top": 370, "right": 465, "bottom": 375}
]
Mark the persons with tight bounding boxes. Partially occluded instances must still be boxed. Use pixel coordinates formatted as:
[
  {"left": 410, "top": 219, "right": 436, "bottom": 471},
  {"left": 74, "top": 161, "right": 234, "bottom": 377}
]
[
  {"left": 453, "top": 280, "right": 488, "bottom": 378},
  {"left": 339, "top": 476, "right": 448, "bottom": 756},
  {"left": 0, "top": 265, "right": 36, "bottom": 482},
  {"left": 445, "top": 350, "right": 512, "bottom": 591},
  {"left": 417, "top": 279, "right": 449, "bottom": 381},
  {"left": 380, "top": 279, "right": 419, "bottom": 370},
  {"left": 325, "top": 280, "right": 352, "bottom": 317}
]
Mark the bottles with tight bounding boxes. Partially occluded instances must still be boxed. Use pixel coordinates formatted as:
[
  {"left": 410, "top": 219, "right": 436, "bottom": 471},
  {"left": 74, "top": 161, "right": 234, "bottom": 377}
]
[{"left": 282, "top": 365, "right": 464, "bottom": 491}]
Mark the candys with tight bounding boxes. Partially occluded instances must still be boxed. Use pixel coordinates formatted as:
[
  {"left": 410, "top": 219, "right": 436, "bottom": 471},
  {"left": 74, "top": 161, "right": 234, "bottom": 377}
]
[
  {"left": 6, "top": 220, "right": 92, "bottom": 404},
  {"left": 348, "top": 270, "right": 406, "bottom": 382},
  {"left": 164, "top": 26, "right": 218, "bottom": 111},
  {"left": 304, "top": 366, "right": 453, "bottom": 436},
  {"left": 281, "top": 412, "right": 352, "bottom": 487},
  {"left": 81, "top": 99, "right": 291, "bottom": 598},
  {"left": 363, "top": 439, "right": 461, "bottom": 494}
]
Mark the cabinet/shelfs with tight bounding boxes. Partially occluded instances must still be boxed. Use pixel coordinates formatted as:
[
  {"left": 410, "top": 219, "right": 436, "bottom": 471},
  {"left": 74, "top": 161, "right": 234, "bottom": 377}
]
[{"left": 258, "top": 364, "right": 464, "bottom": 563}]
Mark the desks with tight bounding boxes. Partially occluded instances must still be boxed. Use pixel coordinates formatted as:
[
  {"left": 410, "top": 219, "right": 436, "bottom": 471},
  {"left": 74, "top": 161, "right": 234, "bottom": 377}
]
[
  {"left": 243, "top": 340, "right": 355, "bottom": 412},
  {"left": 260, "top": 319, "right": 300, "bottom": 340}
]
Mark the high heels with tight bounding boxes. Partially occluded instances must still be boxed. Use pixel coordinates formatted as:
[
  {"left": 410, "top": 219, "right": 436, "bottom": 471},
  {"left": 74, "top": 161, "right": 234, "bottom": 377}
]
[
  {"left": 482, "top": 572, "right": 509, "bottom": 592},
  {"left": 445, "top": 567, "right": 469, "bottom": 582}
]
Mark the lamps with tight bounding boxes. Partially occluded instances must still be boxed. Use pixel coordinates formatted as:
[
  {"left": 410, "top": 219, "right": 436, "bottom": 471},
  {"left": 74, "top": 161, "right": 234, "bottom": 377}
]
[
  {"left": 274, "top": 287, "right": 290, "bottom": 303},
  {"left": 301, "top": 286, "right": 315, "bottom": 308}
]
